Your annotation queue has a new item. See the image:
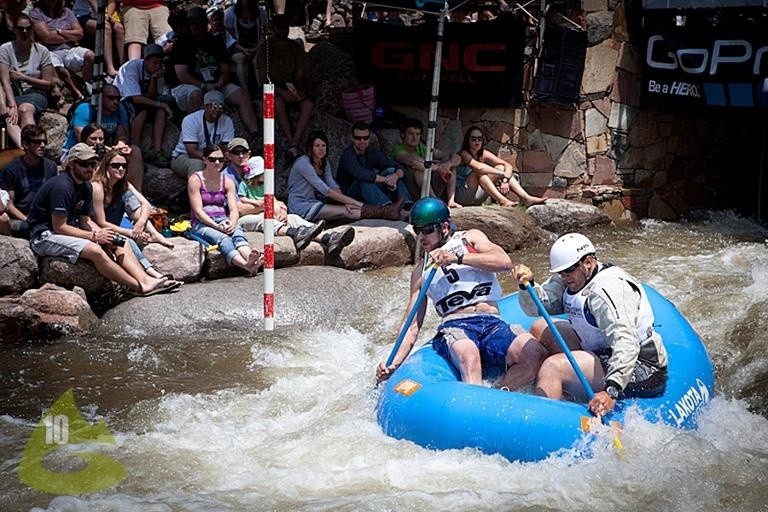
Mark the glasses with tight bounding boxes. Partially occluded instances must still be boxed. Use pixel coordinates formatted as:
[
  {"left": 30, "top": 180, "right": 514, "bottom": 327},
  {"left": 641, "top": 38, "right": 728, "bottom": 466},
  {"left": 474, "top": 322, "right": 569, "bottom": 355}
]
[
  {"left": 412, "top": 220, "right": 444, "bottom": 235},
  {"left": 557, "top": 257, "right": 585, "bottom": 274},
  {"left": 206, "top": 156, "right": 225, "bottom": 162},
  {"left": 27, "top": 138, "right": 48, "bottom": 146},
  {"left": 109, "top": 163, "right": 128, "bottom": 170},
  {"left": 467, "top": 136, "right": 484, "bottom": 142},
  {"left": 228, "top": 149, "right": 248, "bottom": 155},
  {"left": 103, "top": 92, "right": 123, "bottom": 101},
  {"left": 72, "top": 159, "right": 97, "bottom": 169},
  {"left": 352, "top": 135, "right": 371, "bottom": 140},
  {"left": 88, "top": 136, "right": 104, "bottom": 141},
  {"left": 15, "top": 24, "right": 34, "bottom": 32}
]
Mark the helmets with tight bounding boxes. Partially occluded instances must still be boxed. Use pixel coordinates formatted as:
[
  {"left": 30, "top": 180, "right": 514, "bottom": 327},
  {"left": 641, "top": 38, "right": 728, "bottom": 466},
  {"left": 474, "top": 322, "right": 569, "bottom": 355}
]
[
  {"left": 549, "top": 233, "right": 596, "bottom": 281},
  {"left": 408, "top": 196, "right": 451, "bottom": 243}
]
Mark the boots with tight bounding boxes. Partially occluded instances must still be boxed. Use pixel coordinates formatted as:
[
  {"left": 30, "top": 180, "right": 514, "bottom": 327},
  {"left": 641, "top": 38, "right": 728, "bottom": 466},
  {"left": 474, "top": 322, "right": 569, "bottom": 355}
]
[{"left": 360, "top": 196, "right": 404, "bottom": 221}]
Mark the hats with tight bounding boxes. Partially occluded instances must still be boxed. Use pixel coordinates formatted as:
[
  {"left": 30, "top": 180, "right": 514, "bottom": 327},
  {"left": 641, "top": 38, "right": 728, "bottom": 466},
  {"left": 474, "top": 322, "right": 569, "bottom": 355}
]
[
  {"left": 244, "top": 155, "right": 264, "bottom": 180},
  {"left": 183, "top": 6, "right": 208, "bottom": 25},
  {"left": 67, "top": 142, "right": 100, "bottom": 162},
  {"left": 227, "top": 137, "right": 250, "bottom": 151},
  {"left": 143, "top": 44, "right": 165, "bottom": 60},
  {"left": 206, "top": 6, "right": 220, "bottom": 20},
  {"left": 203, "top": 90, "right": 225, "bottom": 105}
]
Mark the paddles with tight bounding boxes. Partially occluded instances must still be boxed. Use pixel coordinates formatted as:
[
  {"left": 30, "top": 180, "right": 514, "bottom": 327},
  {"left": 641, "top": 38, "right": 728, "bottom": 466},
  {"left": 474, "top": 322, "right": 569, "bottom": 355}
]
[
  {"left": 169, "top": 219, "right": 219, "bottom": 252},
  {"left": 519, "top": 270, "right": 628, "bottom": 463}
]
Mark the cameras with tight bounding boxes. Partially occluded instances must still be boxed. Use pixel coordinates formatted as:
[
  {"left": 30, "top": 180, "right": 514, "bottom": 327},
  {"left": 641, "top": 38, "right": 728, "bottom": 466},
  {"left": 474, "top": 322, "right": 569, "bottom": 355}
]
[{"left": 110, "top": 232, "right": 126, "bottom": 246}]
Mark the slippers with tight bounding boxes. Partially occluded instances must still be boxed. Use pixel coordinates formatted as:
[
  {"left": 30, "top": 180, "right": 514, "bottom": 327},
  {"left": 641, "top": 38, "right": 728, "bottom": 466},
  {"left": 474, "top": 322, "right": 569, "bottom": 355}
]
[{"left": 143, "top": 279, "right": 181, "bottom": 296}]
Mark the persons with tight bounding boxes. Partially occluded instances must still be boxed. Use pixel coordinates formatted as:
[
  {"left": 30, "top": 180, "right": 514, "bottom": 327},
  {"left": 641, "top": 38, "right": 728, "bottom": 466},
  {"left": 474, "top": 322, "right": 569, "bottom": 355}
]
[
  {"left": 391, "top": 116, "right": 463, "bottom": 210},
  {"left": 256, "top": 14, "right": 314, "bottom": 164},
  {"left": 104, "top": 1, "right": 124, "bottom": 65},
  {"left": 0, "top": 124, "right": 59, "bottom": 240},
  {"left": 221, "top": 136, "right": 355, "bottom": 258},
  {"left": 67, "top": 1, "right": 118, "bottom": 76},
  {"left": 167, "top": 4, "right": 262, "bottom": 145},
  {"left": 29, "top": 0, "right": 96, "bottom": 102},
  {"left": 477, "top": 5, "right": 498, "bottom": 18},
  {"left": 79, "top": 124, "right": 130, "bottom": 180},
  {"left": 206, "top": 10, "right": 226, "bottom": 46},
  {"left": 221, "top": 0, "right": 268, "bottom": 98},
  {"left": 170, "top": 90, "right": 235, "bottom": 180},
  {"left": 159, "top": 8, "right": 190, "bottom": 46},
  {"left": 0, "top": 0, "right": 27, "bottom": 45},
  {"left": 334, "top": 119, "right": 412, "bottom": 219},
  {"left": 285, "top": 130, "right": 406, "bottom": 222},
  {"left": 110, "top": 43, "right": 174, "bottom": 164},
  {"left": 0, "top": 13, "right": 54, "bottom": 152},
  {"left": 452, "top": 127, "right": 549, "bottom": 209},
  {"left": 25, "top": 142, "right": 184, "bottom": 298},
  {"left": 189, "top": 144, "right": 267, "bottom": 277},
  {"left": 512, "top": 233, "right": 669, "bottom": 418},
  {"left": 101, "top": 0, "right": 172, "bottom": 65},
  {"left": 63, "top": 85, "right": 143, "bottom": 193},
  {"left": 87, "top": 149, "right": 176, "bottom": 287},
  {"left": 375, "top": 197, "right": 549, "bottom": 390},
  {"left": 238, "top": 155, "right": 287, "bottom": 225}
]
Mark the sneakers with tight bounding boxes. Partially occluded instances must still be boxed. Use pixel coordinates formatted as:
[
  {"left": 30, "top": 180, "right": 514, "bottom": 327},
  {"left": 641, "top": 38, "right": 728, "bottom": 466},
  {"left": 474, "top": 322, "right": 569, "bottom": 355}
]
[
  {"left": 326, "top": 226, "right": 355, "bottom": 257},
  {"left": 293, "top": 219, "right": 326, "bottom": 251}
]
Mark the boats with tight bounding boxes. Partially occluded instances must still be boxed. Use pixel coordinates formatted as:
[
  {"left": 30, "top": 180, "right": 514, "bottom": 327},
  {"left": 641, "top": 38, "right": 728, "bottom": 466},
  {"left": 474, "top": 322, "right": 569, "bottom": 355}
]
[{"left": 374, "top": 281, "right": 715, "bottom": 464}]
